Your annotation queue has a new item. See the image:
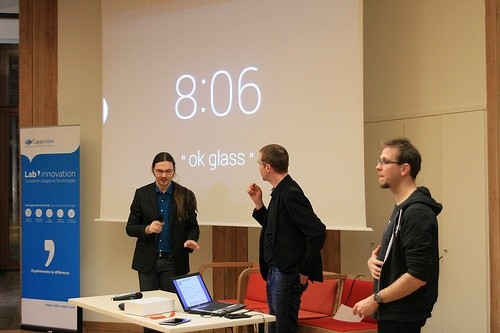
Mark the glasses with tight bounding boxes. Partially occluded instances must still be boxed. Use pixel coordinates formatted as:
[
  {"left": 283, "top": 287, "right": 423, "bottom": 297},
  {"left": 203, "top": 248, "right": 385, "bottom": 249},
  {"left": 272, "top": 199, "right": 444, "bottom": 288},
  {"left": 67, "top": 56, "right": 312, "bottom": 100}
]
[
  {"left": 377, "top": 159, "right": 404, "bottom": 166},
  {"left": 257, "top": 160, "right": 271, "bottom": 167}
]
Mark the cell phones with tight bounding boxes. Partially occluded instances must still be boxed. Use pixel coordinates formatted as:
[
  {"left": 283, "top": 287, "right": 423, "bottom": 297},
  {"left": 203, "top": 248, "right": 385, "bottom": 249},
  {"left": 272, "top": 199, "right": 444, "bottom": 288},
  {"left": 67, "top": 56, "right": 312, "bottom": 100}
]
[{"left": 228, "top": 314, "right": 251, "bottom": 318}]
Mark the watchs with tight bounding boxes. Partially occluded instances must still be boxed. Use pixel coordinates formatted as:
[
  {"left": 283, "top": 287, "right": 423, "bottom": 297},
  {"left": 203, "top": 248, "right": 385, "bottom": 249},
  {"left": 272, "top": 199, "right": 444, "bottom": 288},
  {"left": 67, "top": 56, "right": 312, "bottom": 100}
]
[{"left": 373, "top": 292, "right": 382, "bottom": 307}]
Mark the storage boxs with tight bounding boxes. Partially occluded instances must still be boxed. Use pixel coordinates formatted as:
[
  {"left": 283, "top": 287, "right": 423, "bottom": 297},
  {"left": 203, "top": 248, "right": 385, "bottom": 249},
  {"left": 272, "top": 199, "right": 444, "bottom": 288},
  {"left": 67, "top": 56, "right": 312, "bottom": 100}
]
[{"left": 124, "top": 296, "right": 177, "bottom": 317}]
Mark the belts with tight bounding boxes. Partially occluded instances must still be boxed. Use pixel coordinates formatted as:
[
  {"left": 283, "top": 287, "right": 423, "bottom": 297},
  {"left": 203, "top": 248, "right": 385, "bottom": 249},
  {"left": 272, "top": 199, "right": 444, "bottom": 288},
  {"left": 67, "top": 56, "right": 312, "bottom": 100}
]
[{"left": 158, "top": 252, "right": 173, "bottom": 258}]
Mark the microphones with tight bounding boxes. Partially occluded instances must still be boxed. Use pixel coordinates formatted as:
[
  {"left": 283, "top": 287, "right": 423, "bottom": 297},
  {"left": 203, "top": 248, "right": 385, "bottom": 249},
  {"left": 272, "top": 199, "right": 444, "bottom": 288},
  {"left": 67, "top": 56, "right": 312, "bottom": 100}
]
[{"left": 113, "top": 292, "right": 143, "bottom": 300}]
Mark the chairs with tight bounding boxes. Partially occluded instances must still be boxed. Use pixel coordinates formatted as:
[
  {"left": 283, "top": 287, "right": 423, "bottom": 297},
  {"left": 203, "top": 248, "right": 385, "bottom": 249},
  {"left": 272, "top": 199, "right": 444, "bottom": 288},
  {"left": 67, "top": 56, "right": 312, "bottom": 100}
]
[{"left": 199, "top": 262, "right": 379, "bottom": 333}]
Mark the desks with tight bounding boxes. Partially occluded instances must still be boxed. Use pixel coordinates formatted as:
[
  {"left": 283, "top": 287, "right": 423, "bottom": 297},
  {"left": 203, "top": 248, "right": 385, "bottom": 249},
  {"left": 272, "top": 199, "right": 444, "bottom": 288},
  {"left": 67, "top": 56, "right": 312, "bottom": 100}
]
[{"left": 68, "top": 291, "right": 276, "bottom": 333}]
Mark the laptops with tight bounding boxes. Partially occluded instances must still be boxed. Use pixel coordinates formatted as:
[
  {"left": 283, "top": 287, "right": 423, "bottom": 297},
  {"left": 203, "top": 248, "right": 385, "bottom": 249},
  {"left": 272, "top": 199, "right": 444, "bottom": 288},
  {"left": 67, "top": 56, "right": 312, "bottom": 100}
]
[{"left": 171, "top": 272, "right": 248, "bottom": 316}]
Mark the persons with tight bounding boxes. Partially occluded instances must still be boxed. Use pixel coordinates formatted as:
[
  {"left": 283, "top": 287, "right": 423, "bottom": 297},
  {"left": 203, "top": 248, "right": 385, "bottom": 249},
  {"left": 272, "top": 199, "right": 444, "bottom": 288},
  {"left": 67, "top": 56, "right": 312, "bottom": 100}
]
[
  {"left": 126, "top": 151, "right": 200, "bottom": 333},
  {"left": 247, "top": 144, "right": 326, "bottom": 333},
  {"left": 352, "top": 138, "right": 444, "bottom": 333}
]
